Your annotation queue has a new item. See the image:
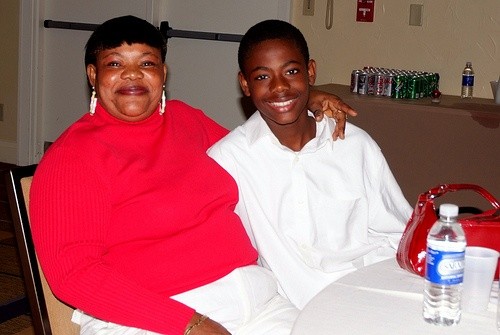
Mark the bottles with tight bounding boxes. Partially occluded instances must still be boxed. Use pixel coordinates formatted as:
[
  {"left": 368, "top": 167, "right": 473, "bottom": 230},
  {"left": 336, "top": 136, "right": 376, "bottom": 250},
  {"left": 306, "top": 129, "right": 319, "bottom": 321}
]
[
  {"left": 422, "top": 204, "right": 467, "bottom": 328},
  {"left": 461, "top": 62, "right": 475, "bottom": 101}
]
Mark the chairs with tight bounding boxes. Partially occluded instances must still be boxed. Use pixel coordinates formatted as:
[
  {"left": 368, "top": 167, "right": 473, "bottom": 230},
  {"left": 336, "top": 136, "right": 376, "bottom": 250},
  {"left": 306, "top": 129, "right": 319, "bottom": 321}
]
[{"left": 7, "top": 163, "right": 81, "bottom": 335}]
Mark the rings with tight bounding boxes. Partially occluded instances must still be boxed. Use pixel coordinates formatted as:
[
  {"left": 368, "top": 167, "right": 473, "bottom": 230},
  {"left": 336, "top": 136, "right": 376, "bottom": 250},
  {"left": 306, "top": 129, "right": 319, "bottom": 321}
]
[{"left": 335, "top": 110, "right": 341, "bottom": 117}]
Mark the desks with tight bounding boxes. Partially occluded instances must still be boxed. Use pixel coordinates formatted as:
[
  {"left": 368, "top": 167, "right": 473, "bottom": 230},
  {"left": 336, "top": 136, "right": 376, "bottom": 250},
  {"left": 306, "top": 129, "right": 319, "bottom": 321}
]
[
  {"left": 291, "top": 258, "right": 500, "bottom": 335},
  {"left": 316, "top": 84, "right": 500, "bottom": 217}
]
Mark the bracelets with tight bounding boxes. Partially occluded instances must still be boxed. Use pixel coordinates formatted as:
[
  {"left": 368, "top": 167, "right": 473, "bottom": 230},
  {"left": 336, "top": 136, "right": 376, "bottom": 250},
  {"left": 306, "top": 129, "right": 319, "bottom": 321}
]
[{"left": 184, "top": 313, "right": 209, "bottom": 335}]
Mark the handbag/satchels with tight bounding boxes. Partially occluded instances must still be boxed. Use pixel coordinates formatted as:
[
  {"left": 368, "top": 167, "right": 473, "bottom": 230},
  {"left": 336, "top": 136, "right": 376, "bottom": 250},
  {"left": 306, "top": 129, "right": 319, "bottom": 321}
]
[{"left": 397, "top": 183, "right": 499, "bottom": 280}]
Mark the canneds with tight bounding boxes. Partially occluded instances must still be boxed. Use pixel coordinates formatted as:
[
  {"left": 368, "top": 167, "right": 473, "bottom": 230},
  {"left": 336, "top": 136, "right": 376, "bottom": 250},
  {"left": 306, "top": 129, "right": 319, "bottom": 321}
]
[{"left": 351, "top": 66, "right": 440, "bottom": 101}]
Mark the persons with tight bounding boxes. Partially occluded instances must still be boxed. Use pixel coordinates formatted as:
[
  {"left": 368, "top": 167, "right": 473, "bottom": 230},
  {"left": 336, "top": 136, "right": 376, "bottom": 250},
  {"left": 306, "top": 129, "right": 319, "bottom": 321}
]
[
  {"left": 206, "top": 18, "right": 413, "bottom": 311},
  {"left": 29, "top": 14, "right": 360, "bottom": 335}
]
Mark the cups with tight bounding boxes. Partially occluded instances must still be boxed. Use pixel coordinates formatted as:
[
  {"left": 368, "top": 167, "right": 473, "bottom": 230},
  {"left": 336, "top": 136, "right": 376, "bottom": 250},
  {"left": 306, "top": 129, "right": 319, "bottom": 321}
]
[
  {"left": 461, "top": 246, "right": 499, "bottom": 314},
  {"left": 490, "top": 81, "right": 500, "bottom": 105}
]
